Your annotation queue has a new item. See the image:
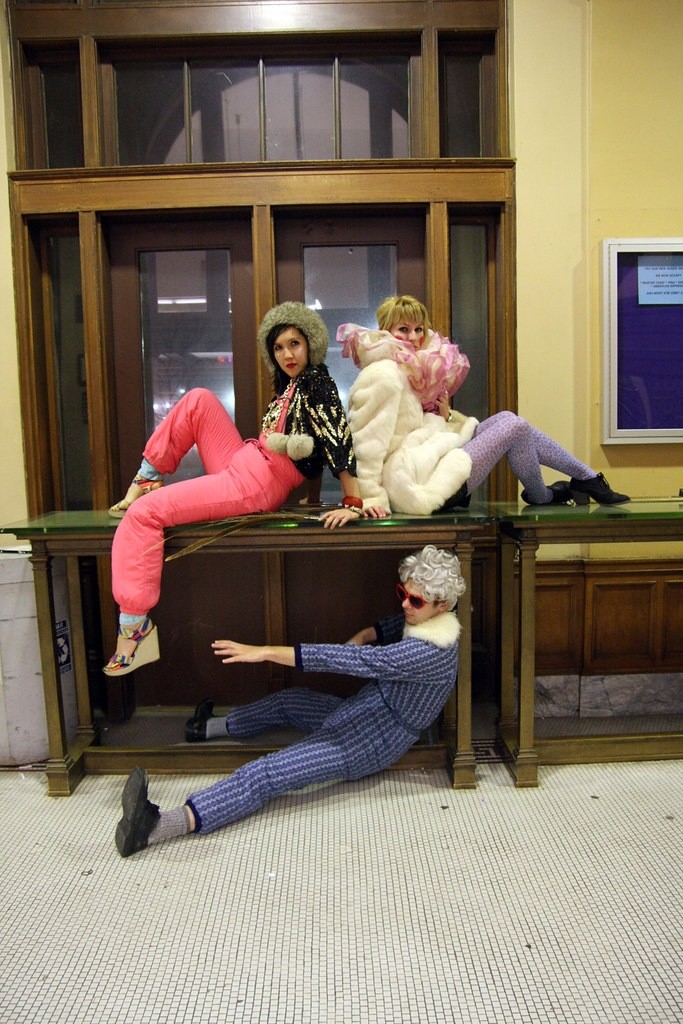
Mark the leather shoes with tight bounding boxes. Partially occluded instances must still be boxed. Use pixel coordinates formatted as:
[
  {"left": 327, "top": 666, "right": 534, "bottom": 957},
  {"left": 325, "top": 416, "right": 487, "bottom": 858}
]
[
  {"left": 184, "top": 698, "right": 215, "bottom": 742},
  {"left": 115, "top": 766, "right": 161, "bottom": 858}
]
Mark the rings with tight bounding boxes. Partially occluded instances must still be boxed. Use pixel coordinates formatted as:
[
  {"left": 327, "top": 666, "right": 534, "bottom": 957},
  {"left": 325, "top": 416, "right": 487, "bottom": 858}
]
[
  {"left": 445, "top": 391, "right": 448, "bottom": 394},
  {"left": 332, "top": 513, "right": 335, "bottom": 516}
]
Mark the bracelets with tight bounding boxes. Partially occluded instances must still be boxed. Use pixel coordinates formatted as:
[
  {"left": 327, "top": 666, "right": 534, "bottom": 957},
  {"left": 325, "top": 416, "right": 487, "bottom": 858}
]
[
  {"left": 341, "top": 496, "right": 363, "bottom": 508},
  {"left": 447, "top": 412, "right": 453, "bottom": 422},
  {"left": 345, "top": 505, "right": 363, "bottom": 515}
]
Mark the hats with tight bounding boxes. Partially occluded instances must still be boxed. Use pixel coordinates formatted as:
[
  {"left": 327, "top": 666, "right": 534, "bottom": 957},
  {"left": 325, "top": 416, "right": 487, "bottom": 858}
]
[{"left": 257, "top": 300, "right": 328, "bottom": 375}]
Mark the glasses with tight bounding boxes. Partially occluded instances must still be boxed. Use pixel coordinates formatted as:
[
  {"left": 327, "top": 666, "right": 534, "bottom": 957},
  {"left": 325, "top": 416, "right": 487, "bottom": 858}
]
[{"left": 396, "top": 582, "right": 427, "bottom": 609}]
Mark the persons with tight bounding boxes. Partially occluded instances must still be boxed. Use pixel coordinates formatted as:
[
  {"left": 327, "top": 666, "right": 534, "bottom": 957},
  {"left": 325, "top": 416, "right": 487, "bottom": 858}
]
[
  {"left": 116, "top": 545, "right": 465, "bottom": 856},
  {"left": 105, "top": 302, "right": 362, "bottom": 675},
  {"left": 338, "top": 294, "right": 630, "bottom": 519}
]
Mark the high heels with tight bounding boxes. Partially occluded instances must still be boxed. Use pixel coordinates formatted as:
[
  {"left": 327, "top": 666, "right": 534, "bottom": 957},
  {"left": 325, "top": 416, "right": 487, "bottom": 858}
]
[
  {"left": 521, "top": 481, "right": 573, "bottom": 505},
  {"left": 102, "top": 617, "right": 160, "bottom": 676},
  {"left": 108, "top": 474, "right": 165, "bottom": 518},
  {"left": 570, "top": 472, "right": 631, "bottom": 507}
]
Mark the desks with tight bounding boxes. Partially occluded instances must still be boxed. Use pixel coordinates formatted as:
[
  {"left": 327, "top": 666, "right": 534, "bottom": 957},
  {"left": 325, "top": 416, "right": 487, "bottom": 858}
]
[
  {"left": 472, "top": 499, "right": 682, "bottom": 788},
  {"left": 1, "top": 509, "right": 499, "bottom": 798}
]
